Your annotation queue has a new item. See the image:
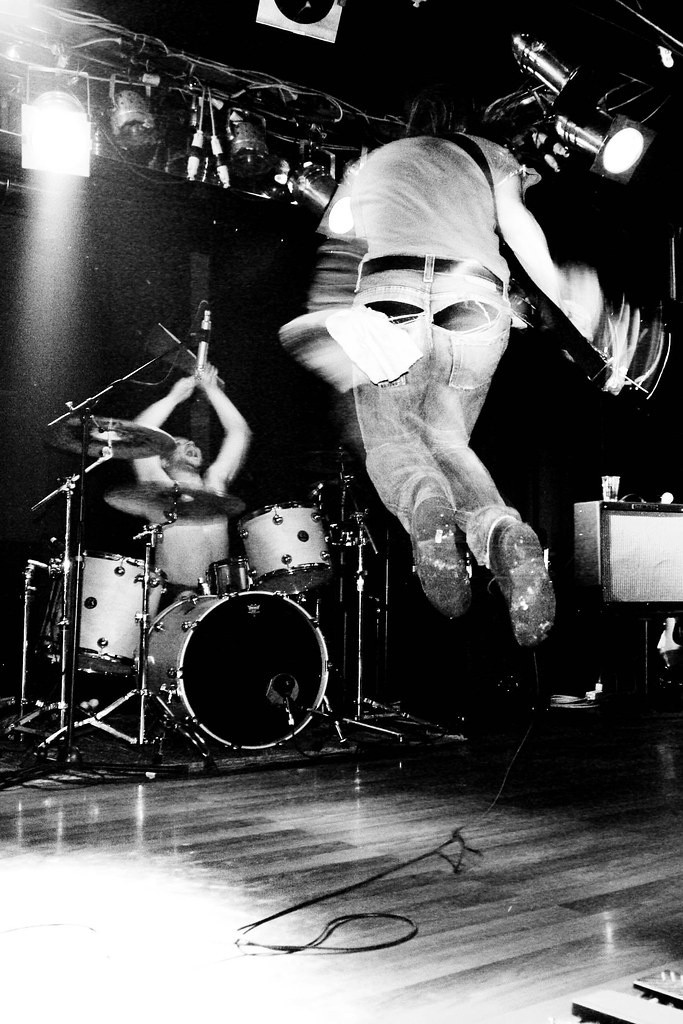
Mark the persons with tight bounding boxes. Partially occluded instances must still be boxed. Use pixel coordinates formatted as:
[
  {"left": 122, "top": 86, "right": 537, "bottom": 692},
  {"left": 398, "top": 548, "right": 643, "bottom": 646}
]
[
  {"left": 292, "top": 98, "right": 604, "bottom": 645},
  {"left": 129, "top": 364, "right": 253, "bottom": 608}
]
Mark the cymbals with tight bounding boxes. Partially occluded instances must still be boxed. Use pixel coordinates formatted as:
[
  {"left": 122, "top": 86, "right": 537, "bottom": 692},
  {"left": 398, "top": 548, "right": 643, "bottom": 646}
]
[
  {"left": 40, "top": 414, "right": 184, "bottom": 462},
  {"left": 102, "top": 483, "right": 245, "bottom": 525},
  {"left": 299, "top": 449, "right": 364, "bottom": 470}
]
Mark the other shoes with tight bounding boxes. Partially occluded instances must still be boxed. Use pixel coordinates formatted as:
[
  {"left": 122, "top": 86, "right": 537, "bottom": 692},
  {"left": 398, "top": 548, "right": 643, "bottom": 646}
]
[
  {"left": 489, "top": 517, "right": 556, "bottom": 646},
  {"left": 411, "top": 492, "right": 472, "bottom": 618}
]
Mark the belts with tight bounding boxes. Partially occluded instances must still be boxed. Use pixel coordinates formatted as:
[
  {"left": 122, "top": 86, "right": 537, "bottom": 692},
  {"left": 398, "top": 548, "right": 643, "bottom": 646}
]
[{"left": 359, "top": 255, "right": 510, "bottom": 292}]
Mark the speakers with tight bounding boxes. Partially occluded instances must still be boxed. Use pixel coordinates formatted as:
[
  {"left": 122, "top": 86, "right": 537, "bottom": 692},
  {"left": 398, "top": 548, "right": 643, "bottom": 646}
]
[{"left": 574, "top": 500, "right": 683, "bottom": 612}]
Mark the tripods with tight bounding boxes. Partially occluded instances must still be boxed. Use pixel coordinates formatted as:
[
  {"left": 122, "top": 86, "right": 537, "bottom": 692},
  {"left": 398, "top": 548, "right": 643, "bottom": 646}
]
[
  {"left": 0, "top": 330, "right": 220, "bottom": 778},
  {"left": 338, "top": 456, "right": 450, "bottom": 739}
]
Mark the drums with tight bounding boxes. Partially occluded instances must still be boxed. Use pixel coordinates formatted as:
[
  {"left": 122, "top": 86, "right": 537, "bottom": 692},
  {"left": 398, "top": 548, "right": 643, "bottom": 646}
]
[
  {"left": 51, "top": 551, "right": 159, "bottom": 675},
  {"left": 236, "top": 500, "right": 334, "bottom": 586},
  {"left": 142, "top": 589, "right": 331, "bottom": 749}
]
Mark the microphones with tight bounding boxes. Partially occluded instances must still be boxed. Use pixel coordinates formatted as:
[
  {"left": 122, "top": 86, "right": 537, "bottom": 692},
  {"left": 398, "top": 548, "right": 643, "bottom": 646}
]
[
  {"left": 196, "top": 310, "right": 212, "bottom": 369},
  {"left": 308, "top": 482, "right": 325, "bottom": 499},
  {"left": 272, "top": 674, "right": 296, "bottom": 702}
]
